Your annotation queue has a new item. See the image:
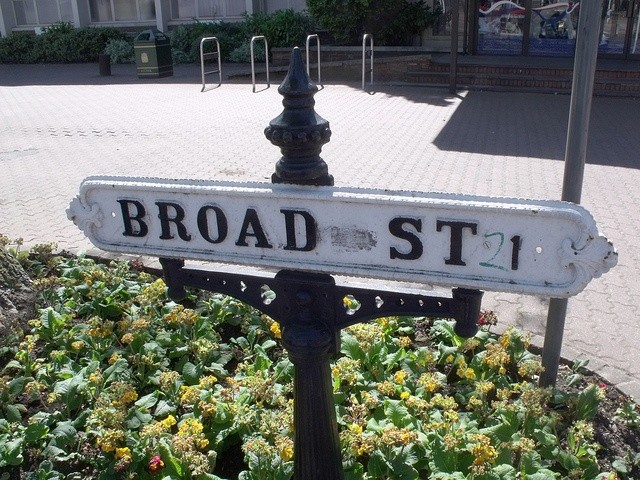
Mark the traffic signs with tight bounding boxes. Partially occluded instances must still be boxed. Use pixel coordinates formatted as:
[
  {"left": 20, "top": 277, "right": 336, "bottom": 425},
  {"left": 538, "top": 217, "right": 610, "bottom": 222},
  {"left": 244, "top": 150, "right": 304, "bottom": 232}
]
[{"left": 64, "top": 177, "right": 618, "bottom": 299}]
